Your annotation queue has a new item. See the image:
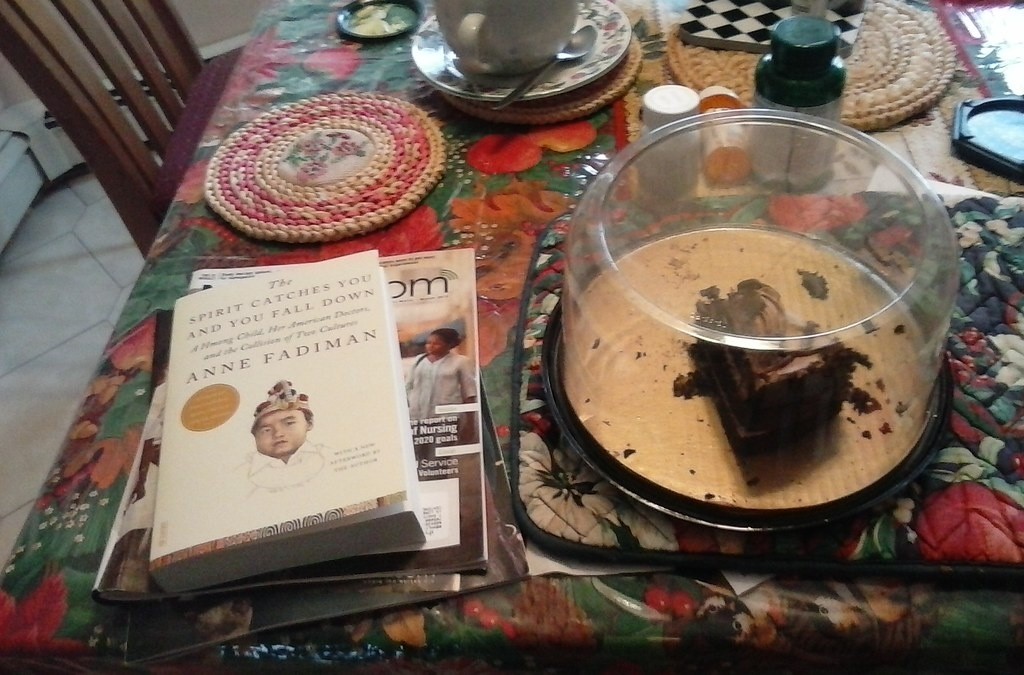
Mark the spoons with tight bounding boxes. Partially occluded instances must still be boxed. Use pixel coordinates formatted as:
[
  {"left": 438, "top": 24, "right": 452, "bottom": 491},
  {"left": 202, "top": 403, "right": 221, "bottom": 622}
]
[{"left": 489, "top": 24, "right": 599, "bottom": 111}]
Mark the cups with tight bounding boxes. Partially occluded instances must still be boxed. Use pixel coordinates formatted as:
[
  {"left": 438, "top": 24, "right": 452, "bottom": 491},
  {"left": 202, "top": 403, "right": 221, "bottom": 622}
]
[{"left": 433, "top": 0, "right": 582, "bottom": 72}]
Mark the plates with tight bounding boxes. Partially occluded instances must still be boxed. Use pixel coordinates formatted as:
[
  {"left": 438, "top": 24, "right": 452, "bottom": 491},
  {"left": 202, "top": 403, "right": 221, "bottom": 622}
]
[
  {"left": 335, "top": 0, "right": 426, "bottom": 40},
  {"left": 410, "top": 0, "right": 632, "bottom": 101}
]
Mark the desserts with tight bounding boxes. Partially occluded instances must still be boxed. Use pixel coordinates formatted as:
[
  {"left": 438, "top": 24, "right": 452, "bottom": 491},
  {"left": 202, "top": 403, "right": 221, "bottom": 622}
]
[{"left": 693, "top": 279, "right": 852, "bottom": 461}]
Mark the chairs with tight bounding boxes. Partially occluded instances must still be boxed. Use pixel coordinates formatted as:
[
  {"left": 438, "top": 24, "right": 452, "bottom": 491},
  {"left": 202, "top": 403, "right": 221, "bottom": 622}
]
[{"left": 0, "top": 1, "right": 247, "bottom": 262}]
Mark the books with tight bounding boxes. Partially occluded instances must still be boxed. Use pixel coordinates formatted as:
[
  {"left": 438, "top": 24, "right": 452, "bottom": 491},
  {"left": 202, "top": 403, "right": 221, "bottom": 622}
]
[
  {"left": 91, "top": 245, "right": 491, "bottom": 608},
  {"left": 149, "top": 250, "right": 427, "bottom": 593},
  {"left": 121, "top": 369, "right": 533, "bottom": 667}
]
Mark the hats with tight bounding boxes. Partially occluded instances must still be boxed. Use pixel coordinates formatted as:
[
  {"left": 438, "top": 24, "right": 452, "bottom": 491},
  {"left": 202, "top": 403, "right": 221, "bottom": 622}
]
[{"left": 251, "top": 378, "right": 313, "bottom": 436}]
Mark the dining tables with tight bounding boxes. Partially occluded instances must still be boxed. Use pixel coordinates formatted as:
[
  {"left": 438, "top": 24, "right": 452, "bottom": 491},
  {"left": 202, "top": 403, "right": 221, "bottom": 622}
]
[{"left": 1, "top": 0, "right": 1024, "bottom": 675}]
